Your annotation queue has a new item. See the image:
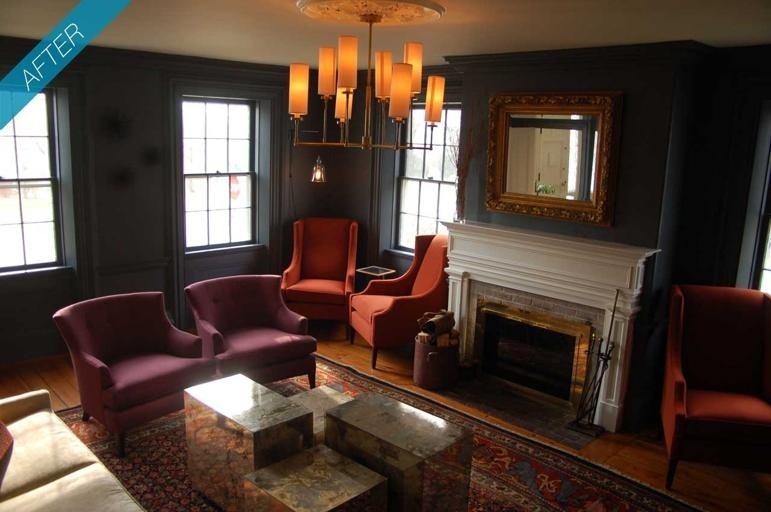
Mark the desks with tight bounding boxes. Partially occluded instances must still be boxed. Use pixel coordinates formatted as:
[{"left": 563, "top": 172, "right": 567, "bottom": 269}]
[{"left": 357, "top": 259, "right": 397, "bottom": 283}]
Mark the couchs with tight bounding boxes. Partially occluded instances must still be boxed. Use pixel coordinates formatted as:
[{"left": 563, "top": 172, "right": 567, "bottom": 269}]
[{"left": 2, "top": 380, "right": 145, "bottom": 511}]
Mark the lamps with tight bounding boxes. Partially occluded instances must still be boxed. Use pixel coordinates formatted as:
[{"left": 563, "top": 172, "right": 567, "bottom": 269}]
[
  {"left": 284, "top": 0, "right": 452, "bottom": 155},
  {"left": 308, "top": 157, "right": 329, "bottom": 186}
]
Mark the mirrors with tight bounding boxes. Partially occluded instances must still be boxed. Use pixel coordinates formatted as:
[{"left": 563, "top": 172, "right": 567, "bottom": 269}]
[{"left": 485, "top": 91, "right": 614, "bottom": 226}]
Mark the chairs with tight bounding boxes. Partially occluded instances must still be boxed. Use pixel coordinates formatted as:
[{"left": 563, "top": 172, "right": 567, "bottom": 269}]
[
  {"left": 280, "top": 214, "right": 361, "bottom": 340},
  {"left": 659, "top": 280, "right": 770, "bottom": 493},
  {"left": 185, "top": 273, "right": 317, "bottom": 390},
  {"left": 351, "top": 231, "right": 455, "bottom": 371},
  {"left": 50, "top": 290, "right": 217, "bottom": 461}
]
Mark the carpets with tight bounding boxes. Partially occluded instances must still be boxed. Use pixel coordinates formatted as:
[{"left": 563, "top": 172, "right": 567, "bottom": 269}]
[{"left": 33, "top": 348, "right": 705, "bottom": 511}]
